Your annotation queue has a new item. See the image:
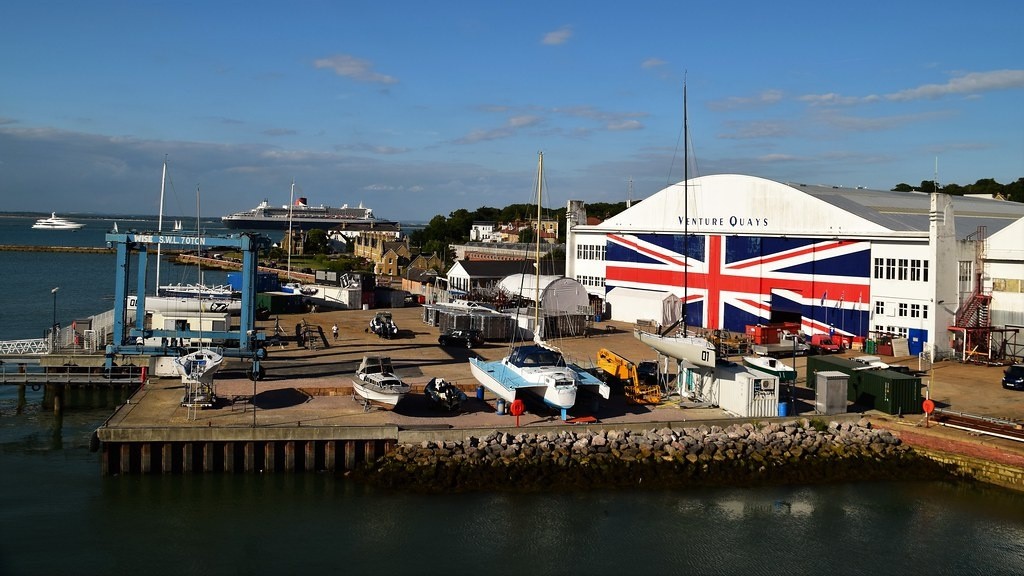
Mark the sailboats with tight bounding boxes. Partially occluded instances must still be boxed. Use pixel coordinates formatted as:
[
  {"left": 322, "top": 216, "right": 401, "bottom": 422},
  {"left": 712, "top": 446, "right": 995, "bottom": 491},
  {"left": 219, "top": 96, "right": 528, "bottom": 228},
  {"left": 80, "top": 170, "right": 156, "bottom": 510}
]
[
  {"left": 126, "top": 155, "right": 364, "bottom": 311},
  {"left": 630, "top": 68, "right": 721, "bottom": 370},
  {"left": 463, "top": 146, "right": 614, "bottom": 408}
]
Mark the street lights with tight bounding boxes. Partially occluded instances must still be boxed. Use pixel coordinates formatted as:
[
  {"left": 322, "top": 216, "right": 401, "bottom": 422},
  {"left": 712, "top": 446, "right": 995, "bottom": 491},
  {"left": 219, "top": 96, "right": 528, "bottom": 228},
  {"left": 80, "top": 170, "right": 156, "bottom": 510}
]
[{"left": 51, "top": 286, "right": 59, "bottom": 327}]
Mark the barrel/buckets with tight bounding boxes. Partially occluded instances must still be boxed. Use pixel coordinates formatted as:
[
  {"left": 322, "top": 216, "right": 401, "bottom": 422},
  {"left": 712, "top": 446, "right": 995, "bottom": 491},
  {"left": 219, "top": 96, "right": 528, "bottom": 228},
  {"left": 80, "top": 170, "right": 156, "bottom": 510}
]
[
  {"left": 596, "top": 315, "right": 601, "bottom": 322},
  {"left": 778, "top": 402, "right": 787, "bottom": 416},
  {"left": 363, "top": 304, "right": 368, "bottom": 310}
]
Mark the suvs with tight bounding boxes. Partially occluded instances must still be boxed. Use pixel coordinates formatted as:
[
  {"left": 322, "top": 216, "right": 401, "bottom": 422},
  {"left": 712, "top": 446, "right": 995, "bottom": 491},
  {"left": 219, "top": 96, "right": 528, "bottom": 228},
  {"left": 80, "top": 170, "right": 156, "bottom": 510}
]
[{"left": 438, "top": 328, "right": 486, "bottom": 351}]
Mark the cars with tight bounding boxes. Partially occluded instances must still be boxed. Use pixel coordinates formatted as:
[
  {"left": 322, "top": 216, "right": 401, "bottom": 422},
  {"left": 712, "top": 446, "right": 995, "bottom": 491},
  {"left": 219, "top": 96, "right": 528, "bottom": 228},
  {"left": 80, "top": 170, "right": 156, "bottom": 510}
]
[
  {"left": 1001, "top": 357, "right": 1024, "bottom": 391},
  {"left": 811, "top": 333, "right": 843, "bottom": 354}
]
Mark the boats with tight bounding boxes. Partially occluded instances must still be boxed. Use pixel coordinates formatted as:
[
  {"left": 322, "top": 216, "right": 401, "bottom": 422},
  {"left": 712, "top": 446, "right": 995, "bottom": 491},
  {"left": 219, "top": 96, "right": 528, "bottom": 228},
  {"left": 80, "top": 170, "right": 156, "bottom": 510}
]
[
  {"left": 370, "top": 312, "right": 398, "bottom": 335},
  {"left": 171, "top": 219, "right": 183, "bottom": 232},
  {"left": 220, "top": 197, "right": 401, "bottom": 228},
  {"left": 352, "top": 354, "right": 410, "bottom": 410},
  {"left": 174, "top": 343, "right": 225, "bottom": 383},
  {"left": 741, "top": 354, "right": 798, "bottom": 382},
  {"left": 31, "top": 212, "right": 87, "bottom": 230},
  {"left": 423, "top": 375, "right": 468, "bottom": 410}
]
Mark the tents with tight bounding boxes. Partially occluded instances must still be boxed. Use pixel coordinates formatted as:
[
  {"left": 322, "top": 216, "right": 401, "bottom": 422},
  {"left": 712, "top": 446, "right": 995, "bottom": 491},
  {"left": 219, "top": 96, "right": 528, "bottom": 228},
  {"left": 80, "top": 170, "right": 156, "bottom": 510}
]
[{"left": 607, "top": 287, "right": 681, "bottom": 327}]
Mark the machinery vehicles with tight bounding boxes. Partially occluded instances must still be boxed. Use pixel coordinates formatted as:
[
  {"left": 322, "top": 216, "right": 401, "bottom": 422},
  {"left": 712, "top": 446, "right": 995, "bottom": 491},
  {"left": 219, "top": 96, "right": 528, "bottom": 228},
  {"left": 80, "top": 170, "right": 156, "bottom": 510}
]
[{"left": 596, "top": 348, "right": 662, "bottom": 405}]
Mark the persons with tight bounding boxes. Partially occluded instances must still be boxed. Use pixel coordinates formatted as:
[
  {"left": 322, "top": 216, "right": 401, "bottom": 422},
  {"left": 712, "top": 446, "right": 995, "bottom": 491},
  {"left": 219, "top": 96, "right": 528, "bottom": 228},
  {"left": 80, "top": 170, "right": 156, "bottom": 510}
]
[
  {"left": 332, "top": 324, "right": 339, "bottom": 342},
  {"left": 283, "top": 297, "right": 320, "bottom": 313},
  {"left": 379, "top": 322, "right": 392, "bottom": 340},
  {"left": 297, "top": 318, "right": 306, "bottom": 333},
  {"left": 432, "top": 292, "right": 438, "bottom": 304},
  {"left": 583, "top": 324, "right": 590, "bottom": 338}
]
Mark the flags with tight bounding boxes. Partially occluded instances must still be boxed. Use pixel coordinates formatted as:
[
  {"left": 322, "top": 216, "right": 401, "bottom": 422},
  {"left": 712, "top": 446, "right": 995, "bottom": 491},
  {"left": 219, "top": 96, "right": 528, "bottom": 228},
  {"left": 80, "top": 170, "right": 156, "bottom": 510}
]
[
  {"left": 839, "top": 291, "right": 844, "bottom": 310},
  {"left": 821, "top": 292, "right": 826, "bottom": 307},
  {"left": 859, "top": 292, "right": 862, "bottom": 310}
]
